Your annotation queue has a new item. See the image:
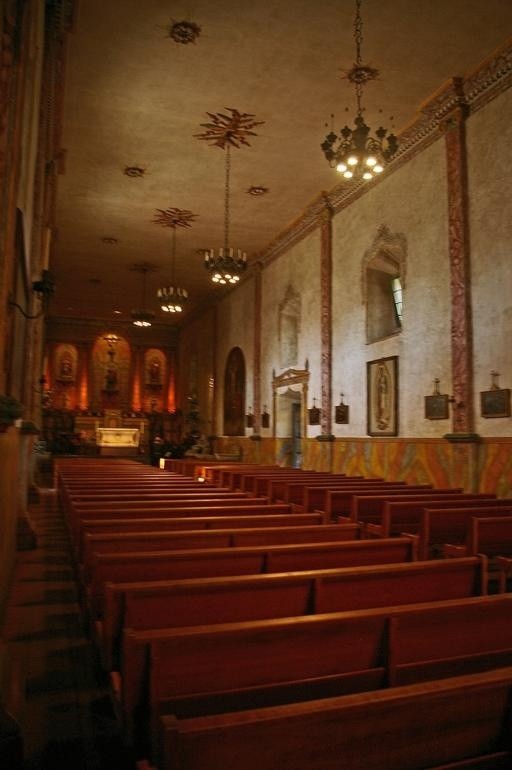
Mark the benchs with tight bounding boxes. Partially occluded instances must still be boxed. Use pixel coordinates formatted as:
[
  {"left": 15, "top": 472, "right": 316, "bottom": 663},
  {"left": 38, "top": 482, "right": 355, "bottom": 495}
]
[{"left": 46, "top": 457, "right": 510, "bottom": 769}]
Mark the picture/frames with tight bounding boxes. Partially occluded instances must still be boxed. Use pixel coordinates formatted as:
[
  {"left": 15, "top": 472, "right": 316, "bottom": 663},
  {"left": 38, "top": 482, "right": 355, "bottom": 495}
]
[{"left": 365, "top": 355, "right": 398, "bottom": 436}]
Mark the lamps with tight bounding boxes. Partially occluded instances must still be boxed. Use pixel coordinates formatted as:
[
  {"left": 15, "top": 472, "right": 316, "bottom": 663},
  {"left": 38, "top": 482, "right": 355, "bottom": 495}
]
[
  {"left": 9, "top": 267, "right": 57, "bottom": 321},
  {"left": 319, "top": 0, "right": 400, "bottom": 182},
  {"left": 196, "top": 105, "right": 266, "bottom": 286},
  {"left": 130, "top": 207, "right": 195, "bottom": 329}
]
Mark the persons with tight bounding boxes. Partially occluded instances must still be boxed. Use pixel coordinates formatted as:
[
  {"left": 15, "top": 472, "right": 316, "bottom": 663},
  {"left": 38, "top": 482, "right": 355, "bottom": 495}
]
[{"left": 147, "top": 435, "right": 175, "bottom": 467}]
